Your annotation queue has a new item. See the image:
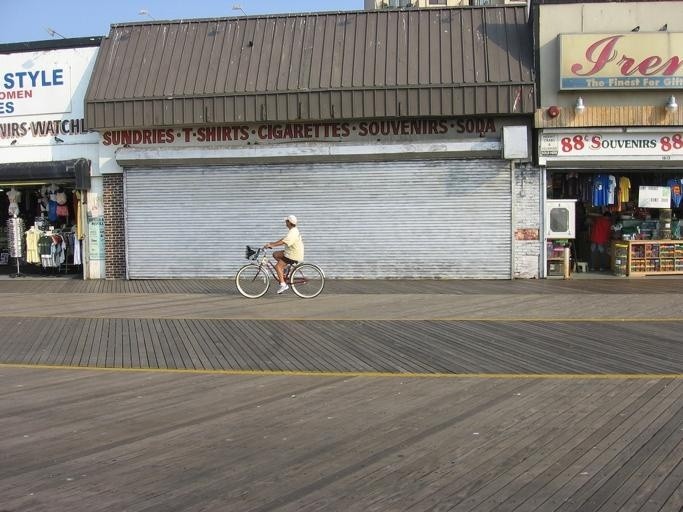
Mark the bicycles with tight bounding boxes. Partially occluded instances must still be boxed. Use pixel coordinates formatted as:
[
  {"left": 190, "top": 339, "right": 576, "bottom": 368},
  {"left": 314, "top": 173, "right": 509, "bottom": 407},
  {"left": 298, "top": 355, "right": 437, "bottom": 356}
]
[{"left": 234, "top": 244, "right": 325, "bottom": 299}]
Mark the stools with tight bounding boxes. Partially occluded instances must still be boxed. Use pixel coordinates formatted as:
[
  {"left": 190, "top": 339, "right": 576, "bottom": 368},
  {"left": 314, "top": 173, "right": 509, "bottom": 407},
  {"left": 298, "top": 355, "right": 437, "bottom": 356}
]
[{"left": 572, "top": 261, "right": 589, "bottom": 273}]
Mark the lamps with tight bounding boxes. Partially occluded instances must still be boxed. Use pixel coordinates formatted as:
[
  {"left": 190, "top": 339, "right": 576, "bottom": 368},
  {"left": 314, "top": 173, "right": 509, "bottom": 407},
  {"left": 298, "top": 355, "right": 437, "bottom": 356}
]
[
  {"left": 137, "top": 9, "right": 157, "bottom": 22},
  {"left": 46, "top": 27, "right": 66, "bottom": 39},
  {"left": 662, "top": 94, "right": 678, "bottom": 114},
  {"left": 231, "top": 3, "right": 247, "bottom": 16},
  {"left": 573, "top": 95, "right": 586, "bottom": 116}
]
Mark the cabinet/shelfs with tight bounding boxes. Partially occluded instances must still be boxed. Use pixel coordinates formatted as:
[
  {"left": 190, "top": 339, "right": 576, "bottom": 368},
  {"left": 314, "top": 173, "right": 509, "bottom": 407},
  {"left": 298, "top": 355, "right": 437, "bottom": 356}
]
[
  {"left": 609, "top": 219, "right": 682, "bottom": 280},
  {"left": 545, "top": 198, "right": 578, "bottom": 279}
]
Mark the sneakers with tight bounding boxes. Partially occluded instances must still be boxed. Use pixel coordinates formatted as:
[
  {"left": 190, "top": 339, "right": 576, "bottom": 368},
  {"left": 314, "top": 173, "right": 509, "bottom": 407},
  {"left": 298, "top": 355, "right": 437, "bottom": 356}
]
[{"left": 277, "top": 284, "right": 289, "bottom": 294}]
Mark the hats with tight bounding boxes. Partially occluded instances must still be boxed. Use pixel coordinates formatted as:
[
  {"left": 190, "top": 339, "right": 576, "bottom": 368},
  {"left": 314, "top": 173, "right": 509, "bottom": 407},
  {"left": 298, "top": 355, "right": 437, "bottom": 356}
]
[{"left": 285, "top": 215, "right": 297, "bottom": 224}]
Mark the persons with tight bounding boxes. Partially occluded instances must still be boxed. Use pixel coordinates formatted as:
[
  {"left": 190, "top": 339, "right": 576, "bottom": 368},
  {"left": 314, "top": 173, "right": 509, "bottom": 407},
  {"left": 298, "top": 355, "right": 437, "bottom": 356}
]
[
  {"left": 7, "top": 217, "right": 25, "bottom": 257},
  {"left": 46, "top": 184, "right": 59, "bottom": 222},
  {"left": 37, "top": 184, "right": 50, "bottom": 211},
  {"left": 7, "top": 187, "right": 22, "bottom": 216},
  {"left": 264, "top": 216, "right": 304, "bottom": 293},
  {"left": 590, "top": 211, "right": 611, "bottom": 273}
]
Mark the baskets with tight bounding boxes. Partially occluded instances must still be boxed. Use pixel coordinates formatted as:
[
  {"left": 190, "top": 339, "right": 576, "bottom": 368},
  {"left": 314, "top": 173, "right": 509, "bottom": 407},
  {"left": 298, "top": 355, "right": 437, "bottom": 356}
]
[{"left": 246, "top": 246, "right": 260, "bottom": 260}]
